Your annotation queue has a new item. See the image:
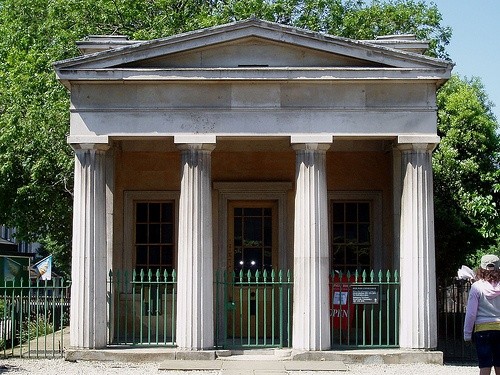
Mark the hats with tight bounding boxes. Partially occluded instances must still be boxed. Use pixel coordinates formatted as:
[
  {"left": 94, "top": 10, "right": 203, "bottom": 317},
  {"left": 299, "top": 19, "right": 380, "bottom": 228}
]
[{"left": 480, "top": 254, "right": 500, "bottom": 271}]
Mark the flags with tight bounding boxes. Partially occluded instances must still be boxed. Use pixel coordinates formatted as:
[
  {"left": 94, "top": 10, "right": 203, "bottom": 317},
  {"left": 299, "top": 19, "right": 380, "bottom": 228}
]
[{"left": 32, "top": 256, "right": 52, "bottom": 281}]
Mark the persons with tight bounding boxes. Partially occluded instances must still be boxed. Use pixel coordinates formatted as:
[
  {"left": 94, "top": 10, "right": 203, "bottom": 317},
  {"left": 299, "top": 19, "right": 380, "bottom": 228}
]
[{"left": 464, "top": 253, "right": 500, "bottom": 375}]
